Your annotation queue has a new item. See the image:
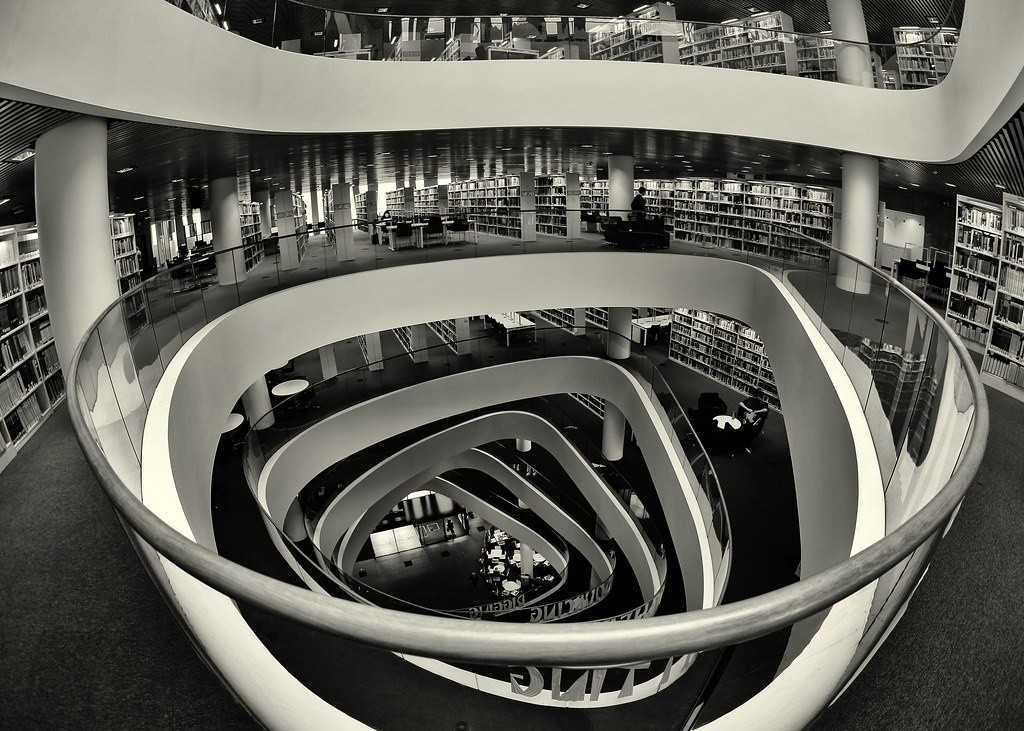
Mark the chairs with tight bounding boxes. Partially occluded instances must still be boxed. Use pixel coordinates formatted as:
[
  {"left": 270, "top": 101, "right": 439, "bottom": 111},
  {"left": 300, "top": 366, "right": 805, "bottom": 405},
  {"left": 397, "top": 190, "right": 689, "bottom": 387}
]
[
  {"left": 900, "top": 257, "right": 924, "bottom": 296},
  {"left": 477, "top": 522, "right": 555, "bottom": 600},
  {"left": 687, "top": 409, "right": 718, "bottom": 457},
  {"left": 737, "top": 400, "right": 769, "bottom": 435},
  {"left": 165, "top": 236, "right": 216, "bottom": 289},
  {"left": 697, "top": 391, "right": 728, "bottom": 416},
  {"left": 485, "top": 310, "right": 538, "bottom": 346},
  {"left": 926, "top": 260, "right": 950, "bottom": 306},
  {"left": 370, "top": 211, "right": 479, "bottom": 252},
  {"left": 723, "top": 424, "right": 756, "bottom": 458}
]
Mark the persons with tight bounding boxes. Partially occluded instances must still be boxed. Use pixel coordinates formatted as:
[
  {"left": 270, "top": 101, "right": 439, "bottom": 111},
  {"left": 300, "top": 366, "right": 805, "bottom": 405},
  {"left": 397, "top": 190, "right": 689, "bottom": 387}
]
[
  {"left": 381, "top": 210, "right": 391, "bottom": 220},
  {"left": 447, "top": 519, "right": 454, "bottom": 533},
  {"left": 631, "top": 187, "right": 646, "bottom": 209}
]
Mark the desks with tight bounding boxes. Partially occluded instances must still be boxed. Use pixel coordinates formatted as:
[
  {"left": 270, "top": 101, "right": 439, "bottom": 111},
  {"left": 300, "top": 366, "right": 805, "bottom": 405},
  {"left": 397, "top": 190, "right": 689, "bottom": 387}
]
[
  {"left": 491, "top": 549, "right": 502, "bottom": 558},
  {"left": 488, "top": 312, "right": 537, "bottom": 347},
  {"left": 883, "top": 256, "right": 952, "bottom": 300},
  {"left": 385, "top": 216, "right": 477, "bottom": 252},
  {"left": 271, "top": 380, "right": 309, "bottom": 396},
  {"left": 512, "top": 553, "right": 521, "bottom": 562},
  {"left": 376, "top": 221, "right": 387, "bottom": 246},
  {"left": 493, "top": 531, "right": 507, "bottom": 541},
  {"left": 220, "top": 413, "right": 244, "bottom": 434},
  {"left": 494, "top": 564, "right": 505, "bottom": 572},
  {"left": 533, "top": 552, "right": 546, "bottom": 561},
  {"left": 503, "top": 580, "right": 519, "bottom": 591},
  {"left": 712, "top": 414, "right": 740, "bottom": 431}
]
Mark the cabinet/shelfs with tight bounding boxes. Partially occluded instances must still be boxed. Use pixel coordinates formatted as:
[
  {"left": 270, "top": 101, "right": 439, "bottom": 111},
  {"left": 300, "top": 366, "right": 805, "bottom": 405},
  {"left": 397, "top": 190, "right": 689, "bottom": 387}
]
[
  {"left": 275, "top": 186, "right": 309, "bottom": 274},
  {"left": 0, "top": 222, "right": 55, "bottom": 454},
  {"left": 448, "top": 177, "right": 478, "bottom": 233},
  {"left": 413, "top": 184, "right": 449, "bottom": 219},
  {"left": 281, "top": 2, "right": 960, "bottom": 90},
  {"left": 355, "top": 331, "right": 385, "bottom": 372},
  {"left": 391, "top": 323, "right": 430, "bottom": 363},
  {"left": 532, "top": 171, "right": 581, "bottom": 240},
  {"left": 475, "top": 172, "right": 497, "bottom": 239},
  {"left": 979, "top": 191, "right": 1024, "bottom": 404},
  {"left": 239, "top": 199, "right": 265, "bottom": 273},
  {"left": 426, "top": 316, "right": 471, "bottom": 356},
  {"left": 353, "top": 190, "right": 378, "bottom": 232},
  {"left": 495, "top": 172, "right": 537, "bottom": 242},
  {"left": 0, "top": 407, "right": 17, "bottom": 474},
  {"left": 568, "top": 174, "right": 881, "bottom": 419},
  {"left": 321, "top": 180, "right": 356, "bottom": 263},
  {"left": 109, "top": 211, "right": 153, "bottom": 340},
  {"left": 318, "top": 343, "right": 339, "bottom": 381},
  {"left": 530, "top": 306, "right": 586, "bottom": 336},
  {"left": 385, "top": 185, "right": 415, "bottom": 218},
  {"left": 15, "top": 225, "right": 68, "bottom": 411},
  {"left": 945, "top": 194, "right": 1004, "bottom": 356}
]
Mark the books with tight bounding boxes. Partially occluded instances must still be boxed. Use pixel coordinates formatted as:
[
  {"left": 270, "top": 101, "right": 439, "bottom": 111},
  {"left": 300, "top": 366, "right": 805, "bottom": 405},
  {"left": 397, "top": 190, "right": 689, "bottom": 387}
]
[
  {"left": 386, "top": 12, "right": 961, "bottom": 90},
  {"left": 0, "top": 174, "right": 1024, "bottom": 472}
]
